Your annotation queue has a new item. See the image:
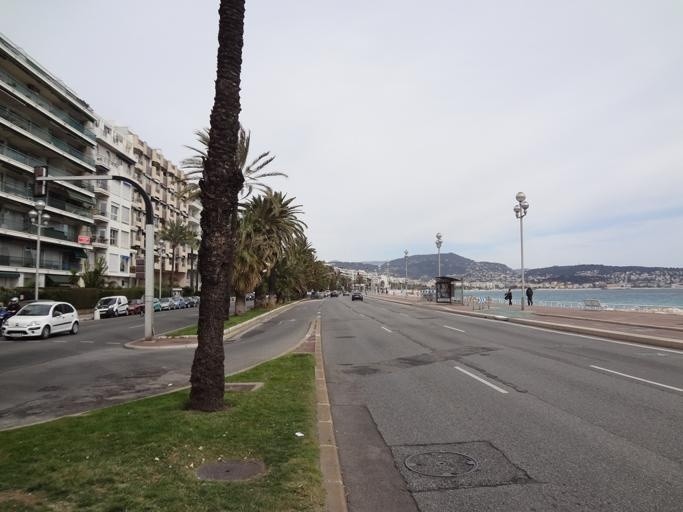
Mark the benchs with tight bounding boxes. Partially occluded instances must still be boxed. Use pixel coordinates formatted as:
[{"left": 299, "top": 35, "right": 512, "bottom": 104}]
[{"left": 583, "top": 300, "right": 608, "bottom": 311}]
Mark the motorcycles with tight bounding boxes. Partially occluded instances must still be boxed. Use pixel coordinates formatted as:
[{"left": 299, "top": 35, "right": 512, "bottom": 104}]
[{"left": 0, "top": 304, "right": 15, "bottom": 332}]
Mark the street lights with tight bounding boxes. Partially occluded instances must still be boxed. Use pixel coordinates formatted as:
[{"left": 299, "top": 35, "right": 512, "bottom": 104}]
[
  {"left": 158, "top": 238, "right": 164, "bottom": 299},
  {"left": 435, "top": 231, "right": 443, "bottom": 277},
  {"left": 403, "top": 248, "right": 409, "bottom": 296},
  {"left": 27, "top": 199, "right": 52, "bottom": 300},
  {"left": 512, "top": 190, "right": 531, "bottom": 310}
]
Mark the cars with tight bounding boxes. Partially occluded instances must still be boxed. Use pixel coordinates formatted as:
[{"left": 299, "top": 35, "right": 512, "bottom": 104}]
[
  {"left": 308, "top": 289, "right": 349, "bottom": 299},
  {"left": 351, "top": 292, "right": 364, "bottom": 302},
  {"left": 93, "top": 293, "right": 200, "bottom": 318},
  {"left": 1, "top": 299, "right": 81, "bottom": 342},
  {"left": 245, "top": 293, "right": 256, "bottom": 301}
]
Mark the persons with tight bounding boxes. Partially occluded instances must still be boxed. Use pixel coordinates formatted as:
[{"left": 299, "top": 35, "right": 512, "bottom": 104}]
[
  {"left": 526, "top": 287, "right": 533, "bottom": 306},
  {"left": 506, "top": 289, "right": 512, "bottom": 306},
  {"left": 6, "top": 296, "right": 21, "bottom": 314},
  {"left": 420, "top": 287, "right": 435, "bottom": 300},
  {"left": 377, "top": 287, "right": 388, "bottom": 294}
]
